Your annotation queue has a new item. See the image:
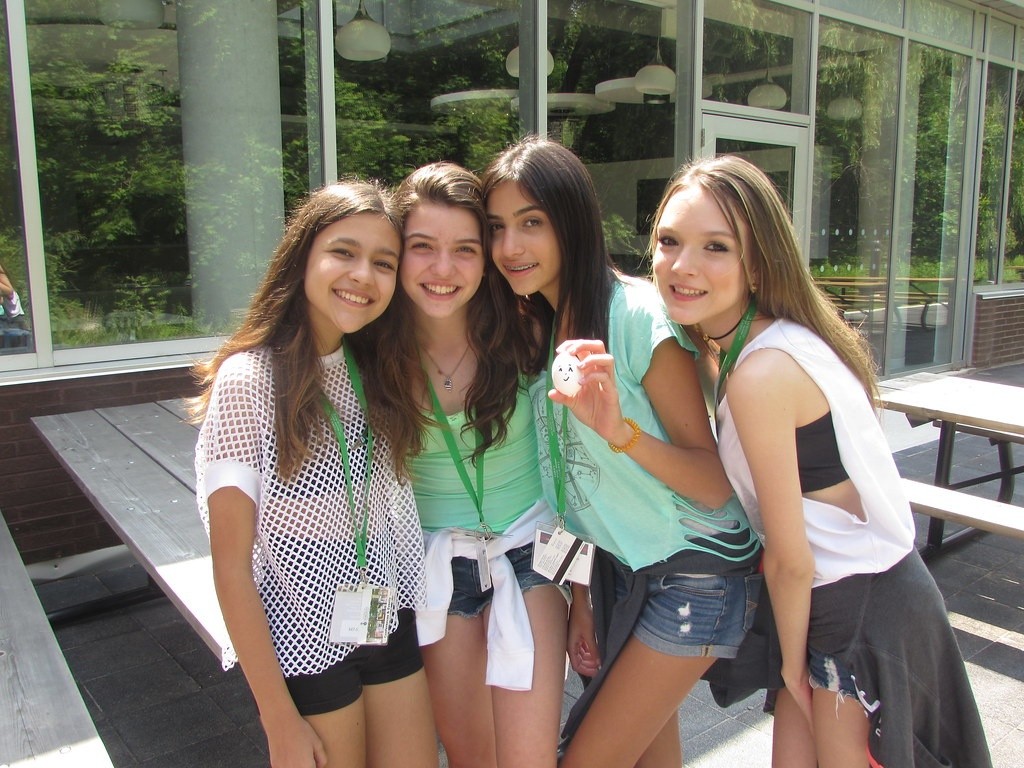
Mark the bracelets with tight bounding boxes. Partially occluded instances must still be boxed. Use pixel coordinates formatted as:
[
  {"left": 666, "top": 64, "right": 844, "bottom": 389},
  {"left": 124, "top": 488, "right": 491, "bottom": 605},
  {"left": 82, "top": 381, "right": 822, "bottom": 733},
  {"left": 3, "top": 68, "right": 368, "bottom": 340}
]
[{"left": 608, "top": 418, "right": 640, "bottom": 453}]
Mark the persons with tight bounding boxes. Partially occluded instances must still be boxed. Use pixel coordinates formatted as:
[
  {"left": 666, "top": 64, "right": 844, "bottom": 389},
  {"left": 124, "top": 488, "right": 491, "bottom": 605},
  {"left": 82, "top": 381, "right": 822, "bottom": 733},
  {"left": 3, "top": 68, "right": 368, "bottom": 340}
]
[
  {"left": 477, "top": 138, "right": 760, "bottom": 767},
  {"left": 0, "top": 265, "right": 25, "bottom": 328},
  {"left": 190, "top": 185, "right": 438, "bottom": 768},
  {"left": 389, "top": 162, "right": 571, "bottom": 768},
  {"left": 653, "top": 156, "right": 994, "bottom": 767}
]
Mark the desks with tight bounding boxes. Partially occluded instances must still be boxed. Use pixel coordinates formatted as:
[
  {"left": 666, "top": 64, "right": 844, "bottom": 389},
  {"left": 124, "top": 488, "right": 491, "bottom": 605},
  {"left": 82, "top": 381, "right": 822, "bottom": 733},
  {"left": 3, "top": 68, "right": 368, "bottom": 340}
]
[
  {"left": 871, "top": 371, "right": 1024, "bottom": 563},
  {"left": 32, "top": 393, "right": 280, "bottom": 669},
  {"left": 812, "top": 274, "right": 955, "bottom": 325}
]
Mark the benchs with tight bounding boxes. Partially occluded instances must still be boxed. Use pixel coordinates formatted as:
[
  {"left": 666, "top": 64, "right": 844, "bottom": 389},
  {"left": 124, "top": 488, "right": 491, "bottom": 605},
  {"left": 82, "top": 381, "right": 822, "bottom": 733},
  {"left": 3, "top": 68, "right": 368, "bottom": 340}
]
[
  {"left": 931, "top": 420, "right": 1024, "bottom": 504},
  {"left": 1, "top": 511, "right": 116, "bottom": 768},
  {"left": 824, "top": 291, "right": 949, "bottom": 330},
  {"left": 898, "top": 475, "right": 1024, "bottom": 533}
]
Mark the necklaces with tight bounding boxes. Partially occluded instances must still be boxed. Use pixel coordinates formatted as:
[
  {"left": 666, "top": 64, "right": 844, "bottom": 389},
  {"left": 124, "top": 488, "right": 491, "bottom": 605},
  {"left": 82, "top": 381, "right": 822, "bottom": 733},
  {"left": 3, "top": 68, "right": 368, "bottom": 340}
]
[
  {"left": 422, "top": 345, "right": 469, "bottom": 391},
  {"left": 704, "top": 314, "right": 747, "bottom": 342}
]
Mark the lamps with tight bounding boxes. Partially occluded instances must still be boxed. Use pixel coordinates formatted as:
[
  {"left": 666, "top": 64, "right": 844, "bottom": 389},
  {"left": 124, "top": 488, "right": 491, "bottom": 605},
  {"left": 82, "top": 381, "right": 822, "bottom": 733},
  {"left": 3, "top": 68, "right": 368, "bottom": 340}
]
[
  {"left": 636, "top": 36, "right": 675, "bottom": 96},
  {"left": 334, "top": 1, "right": 393, "bottom": 62},
  {"left": 506, "top": 39, "right": 555, "bottom": 80},
  {"left": 509, "top": 93, "right": 616, "bottom": 119},
  {"left": 594, "top": 77, "right": 714, "bottom": 106},
  {"left": 746, "top": 34, "right": 788, "bottom": 110},
  {"left": 827, "top": 61, "right": 864, "bottom": 124},
  {"left": 430, "top": 89, "right": 519, "bottom": 112}
]
